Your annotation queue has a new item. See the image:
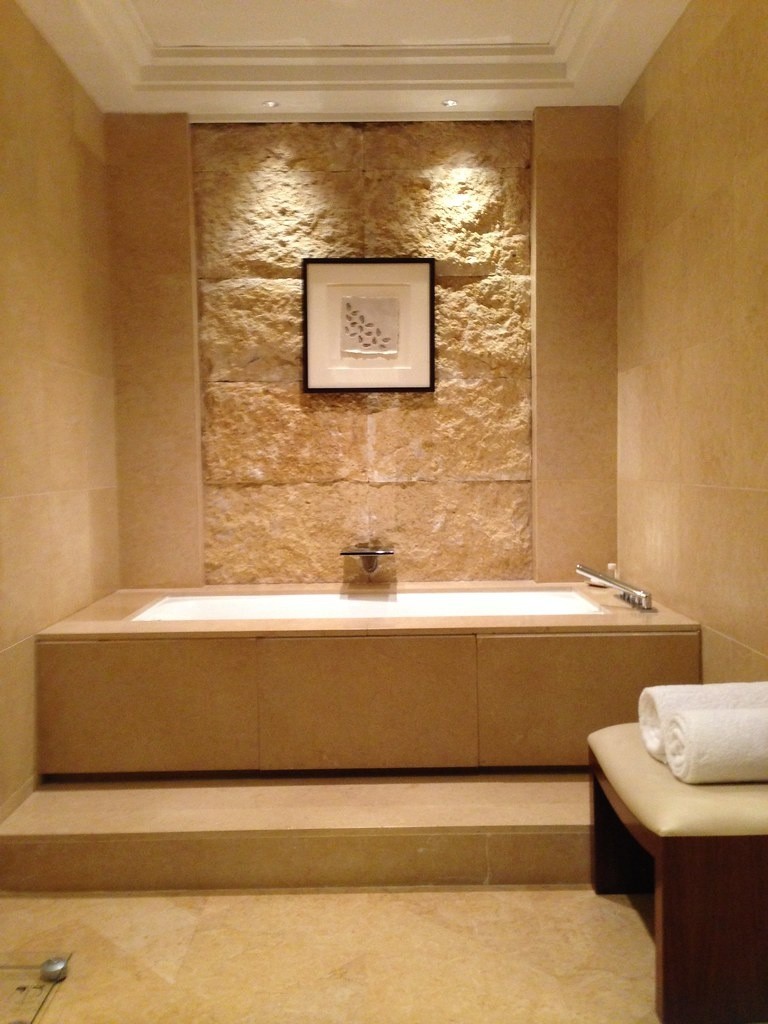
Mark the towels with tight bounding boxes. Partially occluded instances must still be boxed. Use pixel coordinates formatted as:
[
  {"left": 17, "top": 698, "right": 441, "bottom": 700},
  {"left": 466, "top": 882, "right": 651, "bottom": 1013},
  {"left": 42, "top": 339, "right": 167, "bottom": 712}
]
[
  {"left": 638, "top": 680, "right": 768, "bottom": 764},
  {"left": 663, "top": 710, "right": 768, "bottom": 785}
]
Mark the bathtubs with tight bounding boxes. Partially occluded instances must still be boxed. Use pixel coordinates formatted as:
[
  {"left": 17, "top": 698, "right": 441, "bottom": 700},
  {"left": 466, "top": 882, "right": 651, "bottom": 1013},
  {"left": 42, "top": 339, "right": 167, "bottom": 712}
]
[{"left": 33, "top": 589, "right": 702, "bottom": 771}]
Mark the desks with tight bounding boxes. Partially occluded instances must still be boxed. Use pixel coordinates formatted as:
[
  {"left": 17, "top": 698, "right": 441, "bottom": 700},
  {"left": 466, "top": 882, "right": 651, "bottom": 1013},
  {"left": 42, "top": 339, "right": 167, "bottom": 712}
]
[{"left": 586, "top": 747, "right": 768, "bottom": 1024}]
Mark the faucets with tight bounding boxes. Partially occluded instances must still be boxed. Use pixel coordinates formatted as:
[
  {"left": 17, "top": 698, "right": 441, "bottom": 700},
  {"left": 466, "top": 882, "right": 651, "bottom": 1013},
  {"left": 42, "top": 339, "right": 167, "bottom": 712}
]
[
  {"left": 339, "top": 551, "right": 394, "bottom": 571},
  {"left": 575, "top": 563, "right": 652, "bottom": 611}
]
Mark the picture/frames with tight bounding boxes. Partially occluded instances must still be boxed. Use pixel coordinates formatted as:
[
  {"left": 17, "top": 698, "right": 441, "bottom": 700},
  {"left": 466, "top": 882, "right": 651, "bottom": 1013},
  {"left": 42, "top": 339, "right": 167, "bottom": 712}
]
[{"left": 302, "top": 257, "right": 435, "bottom": 393}]
